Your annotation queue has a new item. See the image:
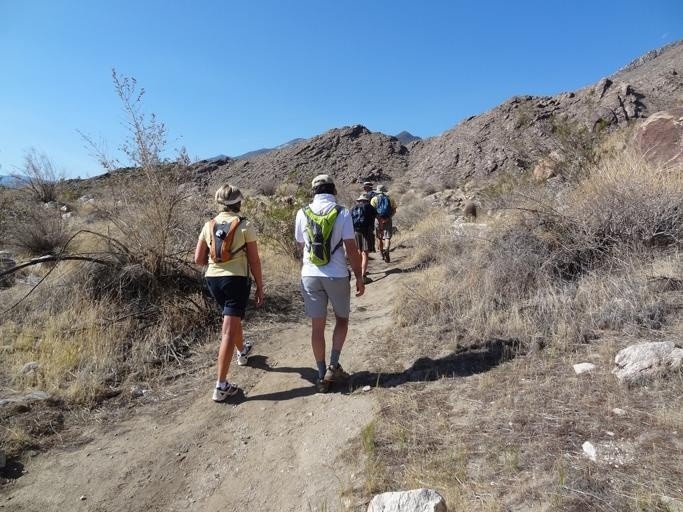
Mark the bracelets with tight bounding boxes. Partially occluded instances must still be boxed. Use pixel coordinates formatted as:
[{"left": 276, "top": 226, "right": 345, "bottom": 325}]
[{"left": 355, "top": 271, "right": 362, "bottom": 279}]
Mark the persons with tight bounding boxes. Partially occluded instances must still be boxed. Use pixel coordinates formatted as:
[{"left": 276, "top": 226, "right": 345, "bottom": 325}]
[
  {"left": 294, "top": 174, "right": 365, "bottom": 393},
  {"left": 194, "top": 182, "right": 264, "bottom": 402},
  {"left": 349, "top": 180, "right": 397, "bottom": 285}
]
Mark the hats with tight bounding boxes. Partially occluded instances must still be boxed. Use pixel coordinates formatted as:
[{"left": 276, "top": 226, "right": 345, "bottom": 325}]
[
  {"left": 375, "top": 185, "right": 387, "bottom": 193},
  {"left": 356, "top": 194, "right": 369, "bottom": 201},
  {"left": 361, "top": 182, "right": 373, "bottom": 188},
  {"left": 216, "top": 193, "right": 245, "bottom": 205},
  {"left": 311, "top": 174, "right": 337, "bottom": 195}
]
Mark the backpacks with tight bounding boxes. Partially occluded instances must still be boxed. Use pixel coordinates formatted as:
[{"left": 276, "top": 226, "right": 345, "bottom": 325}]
[
  {"left": 377, "top": 195, "right": 391, "bottom": 217},
  {"left": 210, "top": 216, "right": 245, "bottom": 262},
  {"left": 302, "top": 205, "right": 343, "bottom": 265},
  {"left": 352, "top": 205, "right": 368, "bottom": 226}
]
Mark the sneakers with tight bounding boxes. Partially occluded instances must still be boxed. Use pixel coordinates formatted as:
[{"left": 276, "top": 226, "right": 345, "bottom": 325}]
[
  {"left": 236, "top": 341, "right": 253, "bottom": 365},
  {"left": 361, "top": 276, "right": 373, "bottom": 284},
  {"left": 214, "top": 381, "right": 239, "bottom": 401},
  {"left": 383, "top": 249, "right": 390, "bottom": 262},
  {"left": 315, "top": 363, "right": 351, "bottom": 393}
]
